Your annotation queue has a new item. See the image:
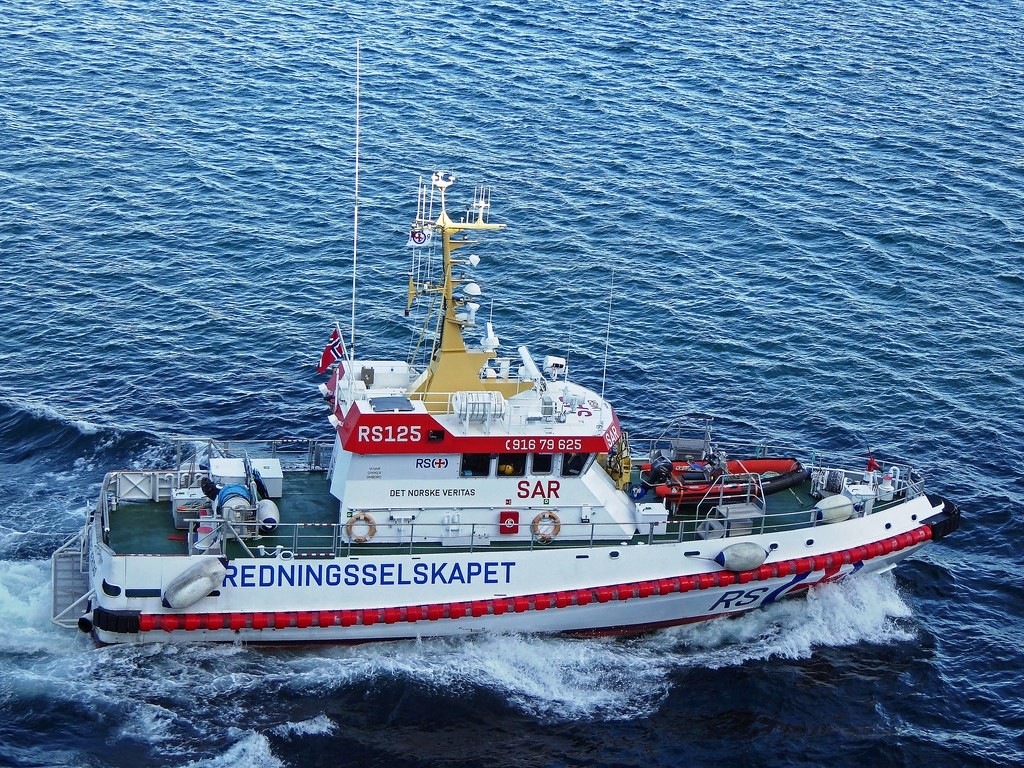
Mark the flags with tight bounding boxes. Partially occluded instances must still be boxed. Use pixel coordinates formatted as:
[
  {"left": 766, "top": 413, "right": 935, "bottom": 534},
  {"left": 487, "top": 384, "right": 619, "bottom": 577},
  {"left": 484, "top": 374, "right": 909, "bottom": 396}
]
[
  {"left": 406, "top": 230, "right": 432, "bottom": 246},
  {"left": 316, "top": 330, "right": 343, "bottom": 374}
]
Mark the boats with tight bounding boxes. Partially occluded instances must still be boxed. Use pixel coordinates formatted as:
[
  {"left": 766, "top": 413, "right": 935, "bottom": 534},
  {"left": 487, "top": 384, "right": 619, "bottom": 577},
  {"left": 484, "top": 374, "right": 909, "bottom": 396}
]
[{"left": 49, "top": 29, "right": 963, "bottom": 644}]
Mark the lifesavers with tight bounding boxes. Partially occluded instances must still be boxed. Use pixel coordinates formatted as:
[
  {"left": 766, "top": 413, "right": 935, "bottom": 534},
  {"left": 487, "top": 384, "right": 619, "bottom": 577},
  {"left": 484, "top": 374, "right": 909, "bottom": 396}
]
[
  {"left": 346, "top": 514, "right": 377, "bottom": 542},
  {"left": 530, "top": 512, "right": 561, "bottom": 542}
]
